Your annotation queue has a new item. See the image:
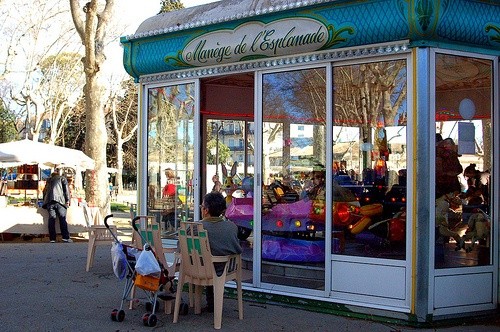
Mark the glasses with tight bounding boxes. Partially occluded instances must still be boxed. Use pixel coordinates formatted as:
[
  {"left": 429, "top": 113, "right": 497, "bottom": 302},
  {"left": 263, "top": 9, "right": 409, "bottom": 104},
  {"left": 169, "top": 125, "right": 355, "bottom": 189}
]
[{"left": 198, "top": 204, "right": 206, "bottom": 208}]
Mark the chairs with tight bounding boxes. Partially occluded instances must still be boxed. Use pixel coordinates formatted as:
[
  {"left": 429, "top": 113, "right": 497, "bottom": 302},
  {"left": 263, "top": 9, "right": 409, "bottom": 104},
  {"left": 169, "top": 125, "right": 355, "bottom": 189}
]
[
  {"left": 131, "top": 216, "right": 194, "bottom": 314},
  {"left": 82, "top": 205, "right": 120, "bottom": 271},
  {"left": 173, "top": 221, "right": 244, "bottom": 330}
]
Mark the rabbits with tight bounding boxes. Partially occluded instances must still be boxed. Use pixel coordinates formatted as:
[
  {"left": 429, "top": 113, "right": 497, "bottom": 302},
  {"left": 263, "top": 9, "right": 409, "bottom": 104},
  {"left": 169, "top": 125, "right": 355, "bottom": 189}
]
[{"left": 219, "top": 161, "right": 238, "bottom": 208}]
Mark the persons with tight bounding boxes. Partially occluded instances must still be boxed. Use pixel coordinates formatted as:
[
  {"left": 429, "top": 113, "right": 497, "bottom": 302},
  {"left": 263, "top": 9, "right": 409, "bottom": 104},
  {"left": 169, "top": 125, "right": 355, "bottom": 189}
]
[
  {"left": 42, "top": 172, "right": 73, "bottom": 243},
  {"left": 212, "top": 175, "right": 222, "bottom": 193},
  {"left": 397, "top": 135, "right": 489, "bottom": 231},
  {"left": 178, "top": 192, "right": 242, "bottom": 311},
  {"left": 162, "top": 168, "right": 175, "bottom": 230}
]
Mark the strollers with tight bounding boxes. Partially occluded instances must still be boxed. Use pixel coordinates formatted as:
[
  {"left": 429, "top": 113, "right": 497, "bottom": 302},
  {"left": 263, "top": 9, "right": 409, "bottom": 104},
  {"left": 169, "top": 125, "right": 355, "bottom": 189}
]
[{"left": 104, "top": 214, "right": 176, "bottom": 327}]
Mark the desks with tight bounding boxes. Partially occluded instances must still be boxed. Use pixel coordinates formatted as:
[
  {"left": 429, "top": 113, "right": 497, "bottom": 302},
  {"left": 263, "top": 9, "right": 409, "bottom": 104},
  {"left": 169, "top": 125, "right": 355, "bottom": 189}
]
[{"left": 0, "top": 206, "right": 103, "bottom": 243}]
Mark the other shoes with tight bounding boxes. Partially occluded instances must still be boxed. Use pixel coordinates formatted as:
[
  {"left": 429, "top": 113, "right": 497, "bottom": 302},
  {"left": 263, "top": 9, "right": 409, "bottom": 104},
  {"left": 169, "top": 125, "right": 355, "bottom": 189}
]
[
  {"left": 61, "top": 239, "right": 74, "bottom": 243},
  {"left": 49, "top": 240, "right": 56, "bottom": 243},
  {"left": 206, "top": 302, "right": 214, "bottom": 312}
]
